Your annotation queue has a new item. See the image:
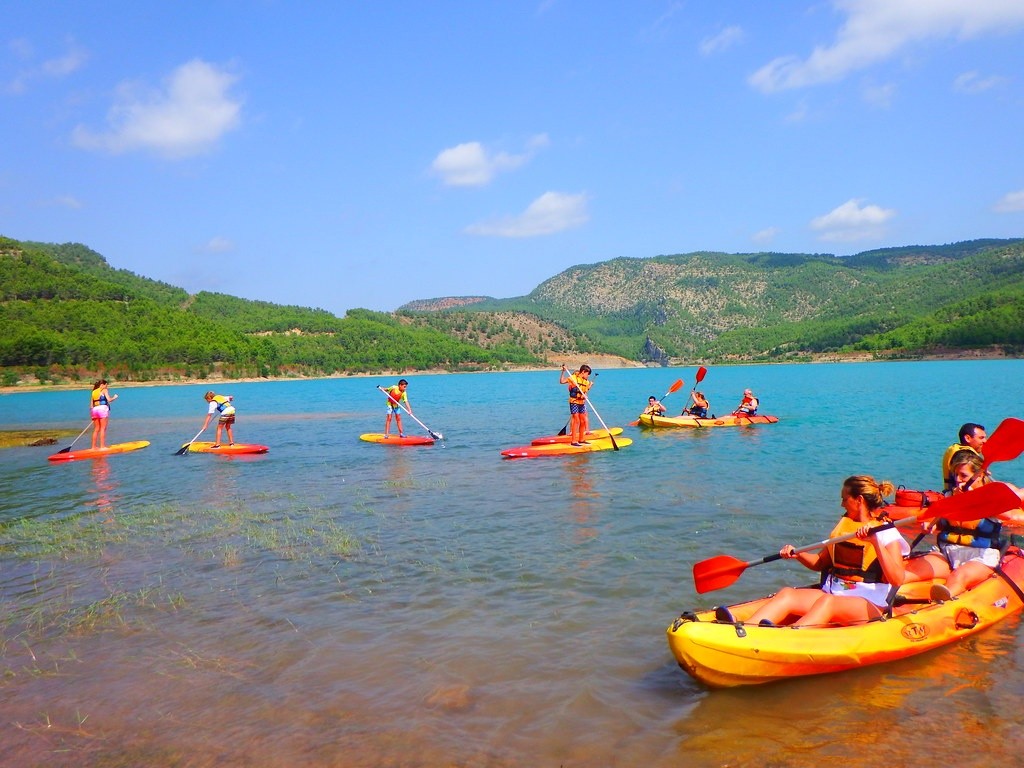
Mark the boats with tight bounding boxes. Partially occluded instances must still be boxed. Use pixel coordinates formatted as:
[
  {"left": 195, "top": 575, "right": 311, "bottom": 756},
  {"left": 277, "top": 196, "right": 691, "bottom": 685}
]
[
  {"left": 640, "top": 413, "right": 778, "bottom": 427},
  {"left": 666, "top": 488, "right": 1024, "bottom": 689}
]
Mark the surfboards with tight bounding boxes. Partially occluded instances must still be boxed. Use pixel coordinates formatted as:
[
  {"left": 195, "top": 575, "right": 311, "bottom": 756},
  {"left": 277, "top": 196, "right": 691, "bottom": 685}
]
[
  {"left": 360, "top": 433, "right": 435, "bottom": 444},
  {"left": 48, "top": 440, "right": 150, "bottom": 461},
  {"left": 501, "top": 436, "right": 632, "bottom": 456},
  {"left": 531, "top": 426, "right": 623, "bottom": 446},
  {"left": 182, "top": 441, "right": 268, "bottom": 453}
]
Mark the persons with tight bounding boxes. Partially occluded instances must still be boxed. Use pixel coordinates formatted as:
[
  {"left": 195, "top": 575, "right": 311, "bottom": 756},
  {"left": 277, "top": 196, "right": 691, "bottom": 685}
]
[
  {"left": 643, "top": 396, "right": 666, "bottom": 417},
  {"left": 733, "top": 388, "right": 759, "bottom": 417},
  {"left": 560, "top": 364, "right": 592, "bottom": 446},
  {"left": 715, "top": 475, "right": 910, "bottom": 629},
  {"left": 201, "top": 391, "right": 236, "bottom": 448},
  {"left": 942, "top": 423, "right": 986, "bottom": 492},
  {"left": 380, "top": 379, "right": 412, "bottom": 439},
  {"left": 903, "top": 450, "right": 1024, "bottom": 602},
  {"left": 682, "top": 390, "right": 709, "bottom": 419},
  {"left": 90, "top": 380, "right": 118, "bottom": 449},
  {"left": 568, "top": 371, "right": 593, "bottom": 436}
]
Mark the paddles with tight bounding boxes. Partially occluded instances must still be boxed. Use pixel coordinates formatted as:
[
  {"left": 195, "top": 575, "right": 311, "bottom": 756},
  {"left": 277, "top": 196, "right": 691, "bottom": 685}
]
[
  {"left": 961, "top": 417, "right": 1024, "bottom": 491},
  {"left": 557, "top": 373, "right": 600, "bottom": 435},
  {"left": 631, "top": 379, "right": 684, "bottom": 426},
  {"left": 691, "top": 480, "right": 1024, "bottom": 593},
  {"left": 177, "top": 410, "right": 221, "bottom": 456},
  {"left": 376, "top": 384, "right": 439, "bottom": 439},
  {"left": 561, "top": 363, "right": 619, "bottom": 451},
  {"left": 734, "top": 396, "right": 752, "bottom": 414},
  {"left": 57, "top": 393, "right": 118, "bottom": 454},
  {"left": 681, "top": 366, "right": 707, "bottom": 416}
]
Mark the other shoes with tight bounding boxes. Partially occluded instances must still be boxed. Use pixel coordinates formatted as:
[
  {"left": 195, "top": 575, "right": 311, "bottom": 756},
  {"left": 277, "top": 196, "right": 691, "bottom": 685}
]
[
  {"left": 385, "top": 435, "right": 389, "bottom": 439},
  {"left": 715, "top": 605, "right": 736, "bottom": 623},
  {"left": 930, "top": 583, "right": 951, "bottom": 600},
  {"left": 585, "top": 431, "right": 592, "bottom": 435},
  {"left": 567, "top": 432, "right": 573, "bottom": 436},
  {"left": 578, "top": 441, "right": 591, "bottom": 445},
  {"left": 571, "top": 442, "right": 583, "bottom": 447},
  {"left": 400, "top": 434, "right": 407, "bottom": 438},
  {"left": 758, "top": 618, "right": 775, "bottom": 626},
  {"left": 229, "top": 442, "right": 234, "bottom": 446},
  {"left": 210, "top": 444, "right": 221, "bottom": 449}
]
